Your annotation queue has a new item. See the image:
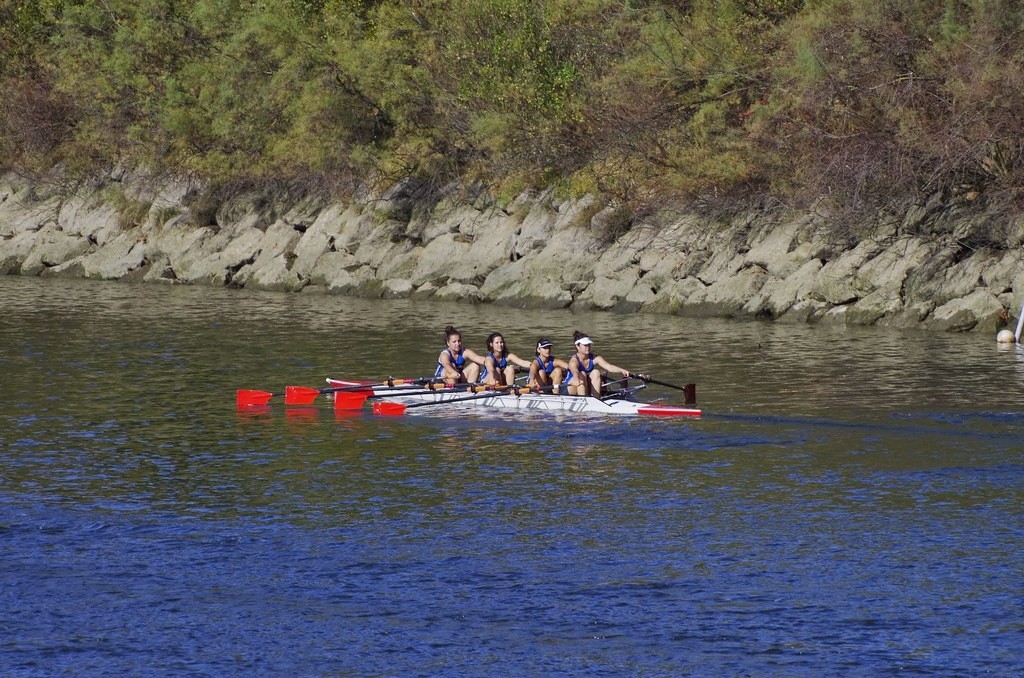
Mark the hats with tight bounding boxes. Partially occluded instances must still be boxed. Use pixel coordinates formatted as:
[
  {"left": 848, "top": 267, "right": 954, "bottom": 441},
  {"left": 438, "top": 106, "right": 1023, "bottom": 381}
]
[
  {"left": 574, "top": 337, "right": 594, "bottom": 345},
  {"left": 537, "top": 339, "right": 553, "bottom": 347}
]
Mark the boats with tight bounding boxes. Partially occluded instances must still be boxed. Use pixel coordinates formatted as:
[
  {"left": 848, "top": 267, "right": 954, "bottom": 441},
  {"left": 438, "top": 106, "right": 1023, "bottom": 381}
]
[{"left": 324, "top": 376, "right": 702, "bottom": 417}]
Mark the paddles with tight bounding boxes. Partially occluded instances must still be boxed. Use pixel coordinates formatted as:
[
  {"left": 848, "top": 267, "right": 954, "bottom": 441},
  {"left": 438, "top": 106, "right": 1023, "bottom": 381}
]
[
  {"left": 627, "top": 372, "right": 696, "bottom": 400},
  {"left": 236, "top": 378, "right": 587, "bottom": 415}
]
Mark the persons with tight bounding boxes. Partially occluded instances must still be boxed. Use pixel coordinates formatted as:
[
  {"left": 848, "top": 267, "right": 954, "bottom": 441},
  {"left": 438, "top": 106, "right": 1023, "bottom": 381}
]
[
  {"left": 559, "top": 330, "right": 630, "bottom": 397},
  {"left": 432, "top": 325, "right": 485, "bottom": 389},
  {"left": 525, "top": 339, "right": 570, "bottom": 395},
  {"left": 479, "top": 331, "right": 533, "bottom": 391}
]
